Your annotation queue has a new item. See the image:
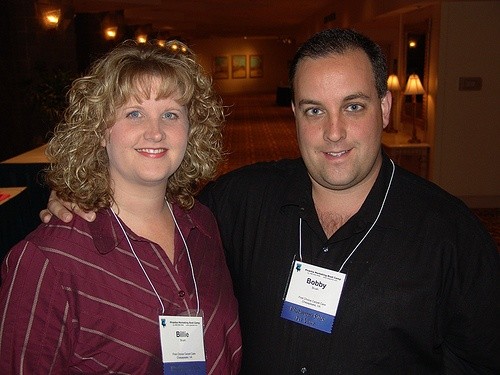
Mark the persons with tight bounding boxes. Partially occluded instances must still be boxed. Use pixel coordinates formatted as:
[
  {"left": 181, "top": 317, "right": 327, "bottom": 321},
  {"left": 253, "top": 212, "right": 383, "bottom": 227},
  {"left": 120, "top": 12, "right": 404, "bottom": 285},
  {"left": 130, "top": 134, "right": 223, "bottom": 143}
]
[
  {"left": 38, "top": 27, "right": 500, "bottom": 375},
  {"left": 0, "top": 40, "right": 241, "bottom": 375}
]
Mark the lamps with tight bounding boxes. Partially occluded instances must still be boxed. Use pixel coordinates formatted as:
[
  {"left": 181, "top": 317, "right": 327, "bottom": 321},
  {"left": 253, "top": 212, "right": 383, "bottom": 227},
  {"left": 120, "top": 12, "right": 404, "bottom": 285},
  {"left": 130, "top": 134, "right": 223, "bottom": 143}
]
[
  {"left": 387, "top": 74, "right": 402, "bottom": 134},
  {"left": 404, "top": 74, "right": 425, "bottom": 144}
]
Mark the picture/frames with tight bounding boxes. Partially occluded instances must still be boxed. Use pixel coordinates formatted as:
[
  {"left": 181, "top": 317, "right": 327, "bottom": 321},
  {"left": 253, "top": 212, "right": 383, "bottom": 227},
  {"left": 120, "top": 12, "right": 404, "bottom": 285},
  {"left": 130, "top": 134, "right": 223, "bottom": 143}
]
[
  {"left": 214, "top": 56, "right": 229, "bottom": 80},
  {"left": 249, "top": 55, "right": 264, "bottom": 79},
  {"left": 231, "top": 54, "right": 247, "bottom": 79}
]
